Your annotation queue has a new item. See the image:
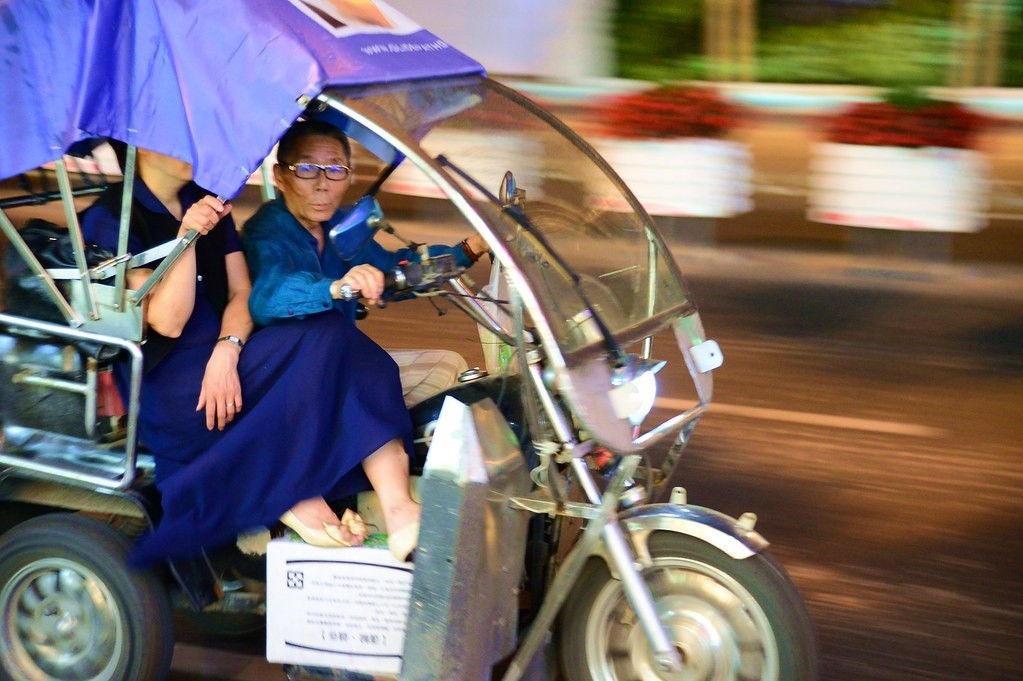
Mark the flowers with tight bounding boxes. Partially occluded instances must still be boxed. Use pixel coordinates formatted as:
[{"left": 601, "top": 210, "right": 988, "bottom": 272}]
[
  {"left": 579, "top": 85, "right": 737, "bottom": 142},
  {"left": 434, "top": 84, "right": 556, "bottom": 132},
  {"left": 817, "top": 88, "right": 988, "bottom": 152}
]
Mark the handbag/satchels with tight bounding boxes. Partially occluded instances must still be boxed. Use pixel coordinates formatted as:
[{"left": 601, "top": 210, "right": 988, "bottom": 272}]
[{"left": 3, "top": 216, "right": 116, "bottom": 359}]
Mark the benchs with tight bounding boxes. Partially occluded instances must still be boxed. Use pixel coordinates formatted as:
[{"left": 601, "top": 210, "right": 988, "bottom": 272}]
[{"left": 0, "top": 185, "right": 467, "bottom": 556}]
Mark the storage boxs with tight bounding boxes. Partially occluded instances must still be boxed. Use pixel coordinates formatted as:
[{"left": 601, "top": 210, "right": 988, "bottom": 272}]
[{"left": 266, "top": 474, "right": 427, "bottom": 677}]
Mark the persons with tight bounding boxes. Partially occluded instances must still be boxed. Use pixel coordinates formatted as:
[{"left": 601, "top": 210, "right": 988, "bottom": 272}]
[
  {"left": 77, "top": 145, "right": 422, "bottom": 566},
  {"left": 244, "top": 122, "right": 511, "bottom": 467}
]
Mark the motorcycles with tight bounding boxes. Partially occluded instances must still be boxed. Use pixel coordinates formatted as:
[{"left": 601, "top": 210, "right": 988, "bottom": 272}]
[{"left": 0, "top": 0, "right": 821, "bottom": 681}]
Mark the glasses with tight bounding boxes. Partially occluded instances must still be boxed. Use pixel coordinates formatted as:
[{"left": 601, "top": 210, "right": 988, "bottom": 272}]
[{"left": 276, "top": 158, "right": 354, "bottom": 180}]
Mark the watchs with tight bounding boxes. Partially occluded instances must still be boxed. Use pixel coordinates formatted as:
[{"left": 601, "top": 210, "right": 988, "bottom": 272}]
[{"left": 214, "top": 335, "right": 244, "bottom": 349}]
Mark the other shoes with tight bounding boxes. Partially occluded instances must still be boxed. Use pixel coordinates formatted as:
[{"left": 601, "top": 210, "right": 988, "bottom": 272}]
[
  {"left": 275, "top": 505, "right": 370, "bottom": 548},
  {"left": 387, "top": 521, "right": 421, "bottom": 561}
]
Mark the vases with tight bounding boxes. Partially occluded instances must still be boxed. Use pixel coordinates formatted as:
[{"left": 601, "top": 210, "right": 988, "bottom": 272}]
[
  {"left": 804, "top": 143, "right": 989, "bottom": 234},
  {"left": 586, "top": 136, "right": 755, "bottom": 219},
  {"left": 383, "top": 132, "right": 547, "bottom": 201}
]
